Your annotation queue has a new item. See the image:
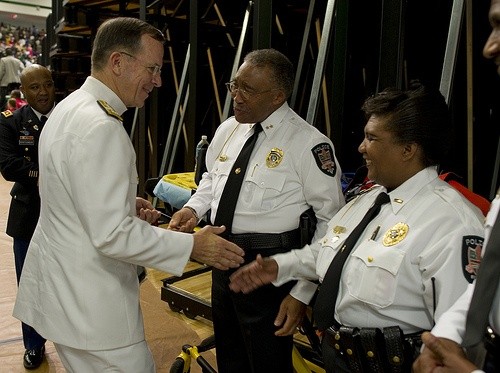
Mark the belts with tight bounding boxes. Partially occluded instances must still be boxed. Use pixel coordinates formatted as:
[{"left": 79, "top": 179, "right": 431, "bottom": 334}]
[
  {"left": 220, "top": 232, "right": 304, "bottom": 249},
  {"left": 482, "top": 323, "right": 500, "bottom": 359},
  {"left": 321, "top": 326, "right": 431, "bottom": 351}
]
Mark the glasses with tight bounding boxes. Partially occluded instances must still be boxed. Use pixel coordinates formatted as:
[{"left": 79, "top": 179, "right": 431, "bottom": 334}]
[
  {"left": 22, "top": 79, "right": 54, "bottom": 92},
  {"left": 225, "top": 79, "right": 286, "bottom": 100},
  {"left": 112, "top": 51, "right": 162, "bottom": 77}
]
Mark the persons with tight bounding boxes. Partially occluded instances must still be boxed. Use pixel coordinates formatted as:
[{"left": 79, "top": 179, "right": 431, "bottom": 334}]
[
  {"left": 229, "top": 93, "right": 486, "bottom": 372},
  {"left": 0, "top": 22, "right": 48, "bottom": 114},
  {"left": 412, "top": 183, "right": 500, "bottom": 373},
  {"left": 0, "top": 64, "right": 65, "bottom": 370},
  {"left": 167, "top": 48, "right": 345, "bottom": 373},
  {"left": 13, "top": 18, "right": 245, "bottom": 373}
]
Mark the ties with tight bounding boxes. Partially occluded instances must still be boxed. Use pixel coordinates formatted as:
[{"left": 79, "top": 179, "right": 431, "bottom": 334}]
[
  {"left": 311, "top": 192, "right": 391, "bottom": 331},
  {"left": 40, "top": 115, "right": 49, "bottom": 126},
  {"left": 459, "top": 208, "right": 500, "bottom": 347},
  {"left": 212, "top": 122, "right": 263, "bottom": 241}
]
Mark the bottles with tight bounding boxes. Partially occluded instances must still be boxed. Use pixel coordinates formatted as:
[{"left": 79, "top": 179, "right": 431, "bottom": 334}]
[{"left": 194, "top": 136, "right": 209, "bottom": 186}]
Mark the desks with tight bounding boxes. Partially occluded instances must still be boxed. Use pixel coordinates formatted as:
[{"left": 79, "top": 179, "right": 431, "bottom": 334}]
[{"left": 152, "top": 171, "right": 198, "bottom": 209}]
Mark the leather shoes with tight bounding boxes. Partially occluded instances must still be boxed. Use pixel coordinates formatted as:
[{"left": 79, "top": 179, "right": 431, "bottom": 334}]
[{"left": 23, "top": 344, "right": 44, "bottom": 369}]
